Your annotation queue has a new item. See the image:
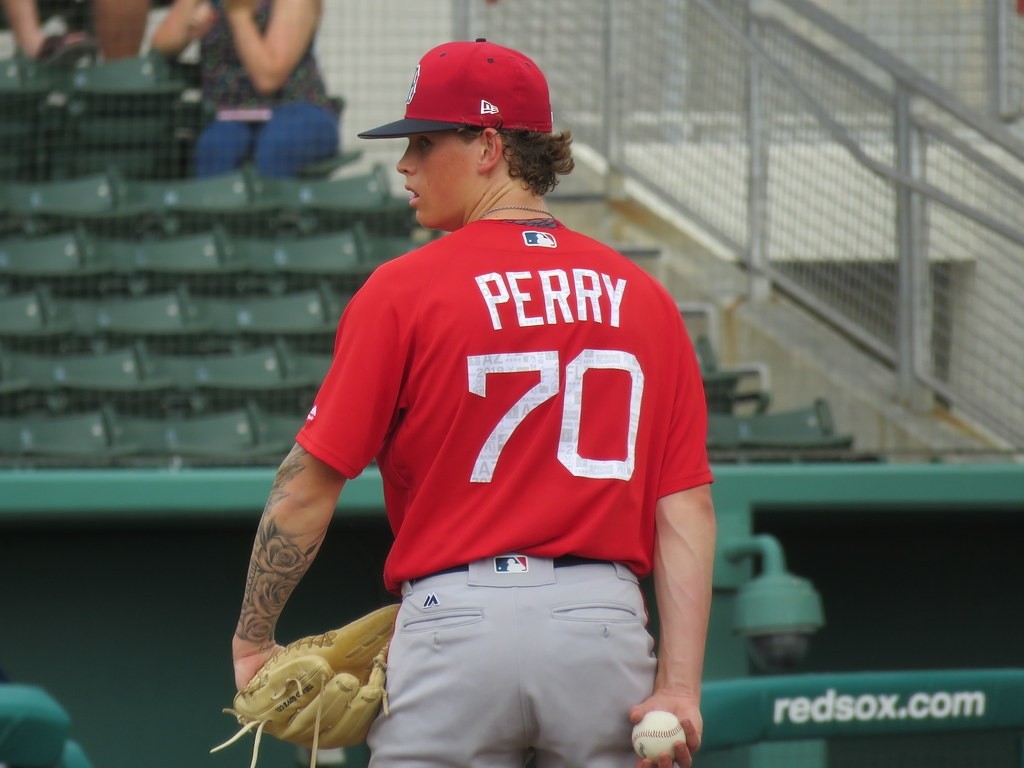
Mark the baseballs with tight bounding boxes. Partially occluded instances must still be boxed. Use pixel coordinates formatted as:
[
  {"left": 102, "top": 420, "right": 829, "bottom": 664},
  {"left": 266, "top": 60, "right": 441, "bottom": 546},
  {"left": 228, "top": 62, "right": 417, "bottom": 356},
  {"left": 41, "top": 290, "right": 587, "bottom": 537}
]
[{"left": 631, "top": 711, "right": 687, "bottom": 764}]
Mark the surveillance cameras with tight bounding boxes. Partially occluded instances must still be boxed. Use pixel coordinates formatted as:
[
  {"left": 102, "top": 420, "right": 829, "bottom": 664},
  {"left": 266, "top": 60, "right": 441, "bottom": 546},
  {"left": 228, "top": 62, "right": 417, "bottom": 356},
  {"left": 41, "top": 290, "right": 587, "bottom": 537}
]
[{"left": 730, "top": 574, "right": 824, "bottom": 669}]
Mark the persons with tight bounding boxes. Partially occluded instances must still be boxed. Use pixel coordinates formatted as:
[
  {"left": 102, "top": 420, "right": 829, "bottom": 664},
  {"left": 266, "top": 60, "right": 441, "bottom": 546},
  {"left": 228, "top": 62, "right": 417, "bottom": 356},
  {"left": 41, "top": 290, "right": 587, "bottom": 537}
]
[
  {"left": 229, "top": 38, "right": 715, "bottom": 768},
  {"left": 149, "top": 0, "right": 345, "bottom": 182},
  {"left": 0, "top": 0, "right": 175, "bottom": 73}
]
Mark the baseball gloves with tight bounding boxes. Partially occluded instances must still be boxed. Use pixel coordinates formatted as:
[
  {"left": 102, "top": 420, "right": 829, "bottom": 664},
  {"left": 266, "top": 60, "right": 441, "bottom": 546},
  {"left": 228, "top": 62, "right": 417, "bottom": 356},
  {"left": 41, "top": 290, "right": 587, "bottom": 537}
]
[{"left": 231, "top": 603, "right": 402, "bottom": 750}]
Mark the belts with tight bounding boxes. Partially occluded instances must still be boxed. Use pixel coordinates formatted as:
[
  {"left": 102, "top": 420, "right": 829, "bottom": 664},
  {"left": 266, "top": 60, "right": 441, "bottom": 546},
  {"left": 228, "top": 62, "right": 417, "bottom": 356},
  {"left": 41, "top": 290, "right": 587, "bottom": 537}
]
[{"left": 414, "top": 551, "right": 613, "bottom": 577}]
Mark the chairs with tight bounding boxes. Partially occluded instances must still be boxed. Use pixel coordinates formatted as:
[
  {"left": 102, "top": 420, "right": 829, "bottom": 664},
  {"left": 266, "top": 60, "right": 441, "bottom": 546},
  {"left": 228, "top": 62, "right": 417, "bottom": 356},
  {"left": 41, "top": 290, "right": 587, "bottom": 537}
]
[{"left": 0, "top": 47, "right": 1024, "bottom": 768}]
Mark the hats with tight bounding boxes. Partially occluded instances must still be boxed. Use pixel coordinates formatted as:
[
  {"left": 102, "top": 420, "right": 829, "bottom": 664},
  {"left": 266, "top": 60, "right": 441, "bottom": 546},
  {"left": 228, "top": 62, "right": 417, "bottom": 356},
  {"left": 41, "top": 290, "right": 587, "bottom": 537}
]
[{"left": 357, "top": 38, "right": 554, "bottom": 139}]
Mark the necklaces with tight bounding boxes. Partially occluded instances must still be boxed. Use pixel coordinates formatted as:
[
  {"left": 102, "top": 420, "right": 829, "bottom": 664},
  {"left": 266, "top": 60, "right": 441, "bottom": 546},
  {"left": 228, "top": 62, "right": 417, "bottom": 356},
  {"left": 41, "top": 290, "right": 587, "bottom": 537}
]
[{"left": 481, "top": 206, "right": 554, "bottom": 219}]
